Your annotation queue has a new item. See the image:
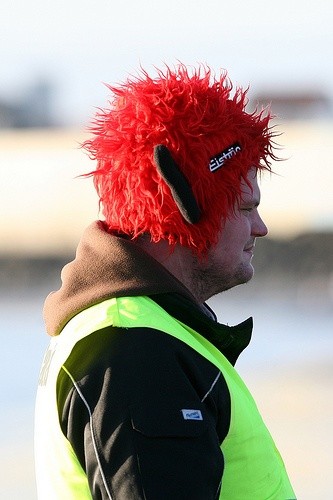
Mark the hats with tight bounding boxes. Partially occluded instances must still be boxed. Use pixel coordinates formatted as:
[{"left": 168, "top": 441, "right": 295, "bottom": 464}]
[{"left": 88, "top": 64, "right": 285, "bottom": 262}]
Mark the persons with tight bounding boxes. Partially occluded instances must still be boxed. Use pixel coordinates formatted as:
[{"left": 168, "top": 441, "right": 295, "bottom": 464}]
[{"left": 28, "top": 56, "right": 306, "bottom": 500}]
